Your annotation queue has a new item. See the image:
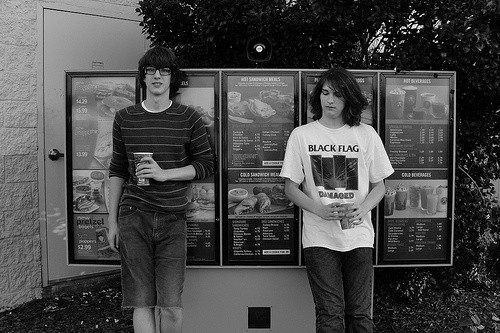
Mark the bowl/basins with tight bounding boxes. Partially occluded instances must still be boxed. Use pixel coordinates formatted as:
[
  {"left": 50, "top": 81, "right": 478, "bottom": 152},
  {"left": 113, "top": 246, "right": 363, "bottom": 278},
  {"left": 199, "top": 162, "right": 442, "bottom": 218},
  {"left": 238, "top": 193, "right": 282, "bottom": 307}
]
[{"left": 228, "top": 188, "right": 248, "bottom": 202}]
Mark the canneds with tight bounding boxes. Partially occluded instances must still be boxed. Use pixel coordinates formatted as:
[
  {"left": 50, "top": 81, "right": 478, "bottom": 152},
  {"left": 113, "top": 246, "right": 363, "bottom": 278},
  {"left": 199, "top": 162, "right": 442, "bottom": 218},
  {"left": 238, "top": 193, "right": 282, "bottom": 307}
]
[{"left": 436, "top": 186, "right": 448, "bottom": 211}]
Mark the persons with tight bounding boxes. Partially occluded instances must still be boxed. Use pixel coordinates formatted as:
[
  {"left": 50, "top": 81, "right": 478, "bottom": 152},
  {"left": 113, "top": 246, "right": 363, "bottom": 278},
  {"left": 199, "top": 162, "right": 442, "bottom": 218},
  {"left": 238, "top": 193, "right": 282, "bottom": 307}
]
[
  {"left": 107, "top": 46, "right": 215, "bottom": 333},
  {"left": 279, "top": 66, "right": 395, "bottom": 333}
]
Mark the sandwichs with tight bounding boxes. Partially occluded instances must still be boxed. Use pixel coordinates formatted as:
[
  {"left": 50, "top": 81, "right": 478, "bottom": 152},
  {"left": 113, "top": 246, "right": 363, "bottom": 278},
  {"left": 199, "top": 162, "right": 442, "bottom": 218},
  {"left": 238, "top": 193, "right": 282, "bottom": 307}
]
[{"left": 72, "top": 194, "right": 96, "bottom": 210}]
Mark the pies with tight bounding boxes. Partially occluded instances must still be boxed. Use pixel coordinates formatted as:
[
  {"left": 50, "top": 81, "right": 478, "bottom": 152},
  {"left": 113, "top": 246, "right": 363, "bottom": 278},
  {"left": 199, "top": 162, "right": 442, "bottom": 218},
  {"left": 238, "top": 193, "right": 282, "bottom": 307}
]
[{"left": 234, "top": 192, "right": 271, "bottom": 215}]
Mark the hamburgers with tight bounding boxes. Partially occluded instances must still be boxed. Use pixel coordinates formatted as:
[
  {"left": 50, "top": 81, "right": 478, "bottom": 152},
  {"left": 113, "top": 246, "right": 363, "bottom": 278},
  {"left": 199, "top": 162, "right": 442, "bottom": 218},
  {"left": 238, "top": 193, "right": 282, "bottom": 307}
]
[{"left": 93, "top": 84, "right": 136, "bottom": 116}]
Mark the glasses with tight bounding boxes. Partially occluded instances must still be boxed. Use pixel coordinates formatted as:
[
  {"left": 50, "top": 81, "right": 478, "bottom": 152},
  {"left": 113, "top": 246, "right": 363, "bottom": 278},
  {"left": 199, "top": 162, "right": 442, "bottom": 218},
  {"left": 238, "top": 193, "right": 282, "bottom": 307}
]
[{"left": 143, "top": 66, "right": 171, "bottom": 75}]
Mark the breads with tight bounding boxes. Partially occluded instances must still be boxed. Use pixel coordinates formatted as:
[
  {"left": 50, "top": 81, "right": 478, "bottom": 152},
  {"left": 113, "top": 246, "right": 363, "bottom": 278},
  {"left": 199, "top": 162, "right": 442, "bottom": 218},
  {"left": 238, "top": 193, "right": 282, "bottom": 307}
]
[
  {"left": 228, "top": 98, "right": 276, "bottom": 119},
  {"left": 198, "top": 184, "right": 214, "bottom": 201}
]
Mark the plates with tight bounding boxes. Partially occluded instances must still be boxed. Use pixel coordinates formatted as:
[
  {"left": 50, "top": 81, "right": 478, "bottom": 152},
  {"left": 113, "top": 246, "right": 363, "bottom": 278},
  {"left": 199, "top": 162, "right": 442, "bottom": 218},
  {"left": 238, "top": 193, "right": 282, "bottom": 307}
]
[{"left": 73, "top": 204, "right": 100, "bottom": 213}]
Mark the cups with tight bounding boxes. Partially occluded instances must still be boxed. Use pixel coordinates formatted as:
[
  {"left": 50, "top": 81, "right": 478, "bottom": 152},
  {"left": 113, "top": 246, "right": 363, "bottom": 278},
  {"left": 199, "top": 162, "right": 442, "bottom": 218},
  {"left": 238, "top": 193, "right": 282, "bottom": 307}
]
[
  {"left": 395, "top": 189, "right": 407, "bottom": 210},
  {"left": 436, "top": 187, "right": 447, "bottom": 212},
  {"left": 385, "top": 189, "right": 396, "bottom": 216},
  {"left": 336, "top": 203, "right": 356, "bottom": 229},
  {"left": 421, "top": 187, "right": 434, "bottom": 211},
  {"left": 389, "top": 86, "right": 448, "bottom": 120},
  {"left": 409, "top": 190, "right": 419, "bottom": 208},
  {"left": 426, "top": 193, "right": 438, "bottom": 215},
  {"left": 133, "top": 152, "right": 153, "bottom": 186},
  {"left": 228, "top": 92, "right": 241, "bottom": 102}
]
